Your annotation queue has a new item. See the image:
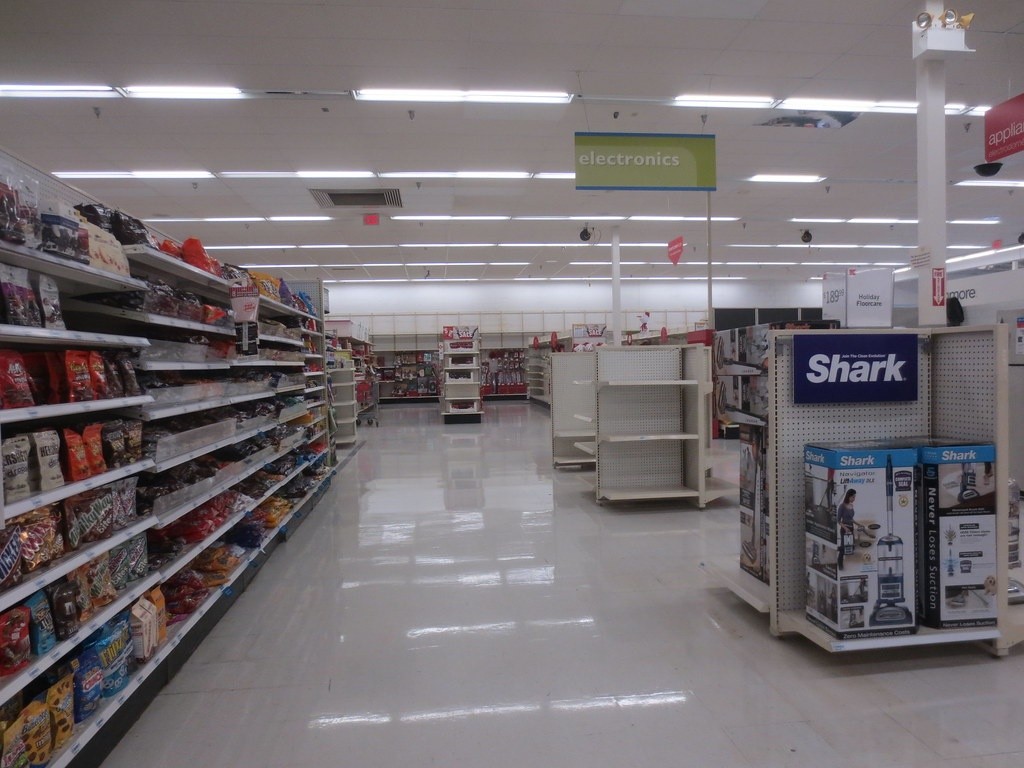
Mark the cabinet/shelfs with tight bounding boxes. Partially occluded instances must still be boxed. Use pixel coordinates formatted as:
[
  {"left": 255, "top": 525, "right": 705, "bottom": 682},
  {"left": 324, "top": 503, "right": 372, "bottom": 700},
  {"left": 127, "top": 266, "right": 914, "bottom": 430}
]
[
  {"left": 695, "top": 327, "right": 1010, "bottom": 653},
  {"left": 325, "top": 335, "right": 437, "bottom": 444},
  {"left": 0, "top": 243, "right": 332, "bottom": 768},
  {"left": 572, "top": 342, "right": 739, "bottom": 514},
  {"left": 528, "top": 338, "right": 550, "bottom": 409},
  {"left": 438, "top": 341, "right": 484, "bottom": 425}
]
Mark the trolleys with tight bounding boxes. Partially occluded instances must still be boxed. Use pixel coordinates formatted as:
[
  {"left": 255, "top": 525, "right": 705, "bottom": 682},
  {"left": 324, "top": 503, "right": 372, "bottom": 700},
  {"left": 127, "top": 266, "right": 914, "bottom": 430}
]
[{"left": 356, "top": 374, "right": 382, "bottom": 427}]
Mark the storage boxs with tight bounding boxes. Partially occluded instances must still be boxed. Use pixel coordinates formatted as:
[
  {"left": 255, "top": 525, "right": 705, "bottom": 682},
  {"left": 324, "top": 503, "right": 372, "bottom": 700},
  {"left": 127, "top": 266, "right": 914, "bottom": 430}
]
[
  {"left": 711, "top": 320, "right": 996, "bottom": 639},
  {"left": 716, "top": 326, "right": 997, "bottom": 638}
]
